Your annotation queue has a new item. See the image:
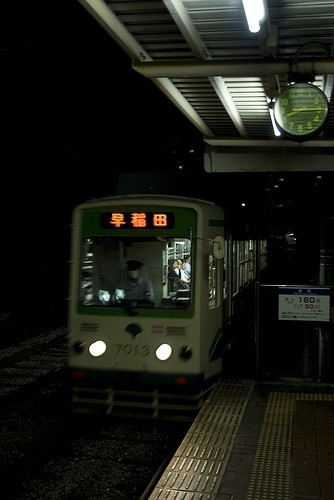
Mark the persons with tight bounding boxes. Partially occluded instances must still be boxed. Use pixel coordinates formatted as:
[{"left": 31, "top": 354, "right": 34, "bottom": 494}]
[
  {"left": 169, "top": 255, "right": 192, "bottom": 291},
  {"left": 113, "top": 261, "right": 154, "bottom": 305}
]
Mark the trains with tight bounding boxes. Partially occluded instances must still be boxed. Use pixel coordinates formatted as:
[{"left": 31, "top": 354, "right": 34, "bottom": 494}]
[{"left": 65, "top": 192, "right": 268, "bottom": 412}]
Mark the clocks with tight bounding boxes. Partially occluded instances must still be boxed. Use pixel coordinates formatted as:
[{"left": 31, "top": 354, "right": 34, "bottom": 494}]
[{"left": 273, "top": 83, "right": 330, "bottom": 141}]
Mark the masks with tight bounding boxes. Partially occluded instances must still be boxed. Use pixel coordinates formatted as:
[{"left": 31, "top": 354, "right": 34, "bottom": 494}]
[{"left": 127, "top": 270, "right": 142, "bottom": 279}]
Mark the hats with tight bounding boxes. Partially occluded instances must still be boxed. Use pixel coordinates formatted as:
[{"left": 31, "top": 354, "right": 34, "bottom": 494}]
[{"left": 126, "top": 260, "right": 144, "bottom": 266}]
